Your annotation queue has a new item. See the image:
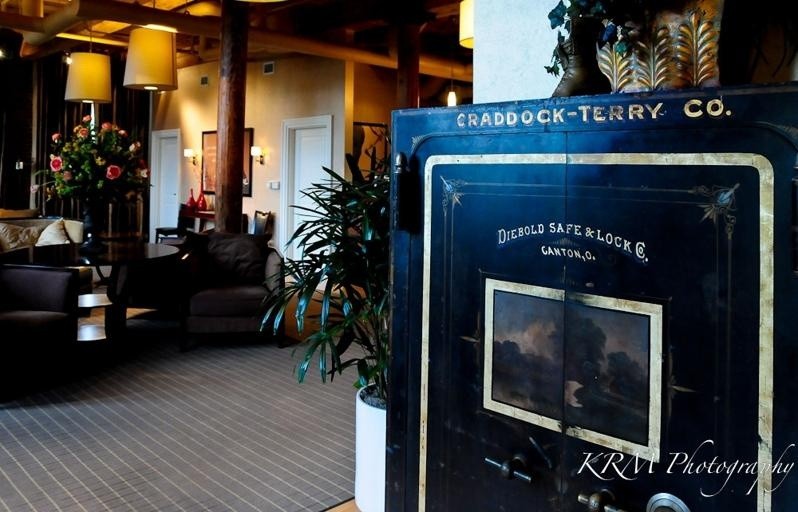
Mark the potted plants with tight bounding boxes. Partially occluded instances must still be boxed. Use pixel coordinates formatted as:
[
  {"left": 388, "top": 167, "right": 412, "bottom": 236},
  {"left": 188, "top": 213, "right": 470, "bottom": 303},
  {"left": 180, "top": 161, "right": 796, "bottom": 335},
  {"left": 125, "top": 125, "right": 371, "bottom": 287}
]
[{"left": 258, "top": 121, "right": 391, "bottom": 512}]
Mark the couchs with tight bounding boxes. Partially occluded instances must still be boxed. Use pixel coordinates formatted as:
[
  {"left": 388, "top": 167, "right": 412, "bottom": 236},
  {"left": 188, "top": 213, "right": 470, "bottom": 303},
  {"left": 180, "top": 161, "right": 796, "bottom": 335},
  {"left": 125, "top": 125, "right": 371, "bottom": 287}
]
[{"left": 0, "top": 217, "right": 84, "bottom": 254}]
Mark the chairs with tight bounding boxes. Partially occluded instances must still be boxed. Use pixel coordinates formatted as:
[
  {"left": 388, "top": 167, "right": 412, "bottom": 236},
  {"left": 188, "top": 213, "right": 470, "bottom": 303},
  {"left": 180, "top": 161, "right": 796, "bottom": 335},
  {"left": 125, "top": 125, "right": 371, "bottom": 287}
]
[
  {"left": 0, "top": 264, "right": 79, "bottom": 404},
  {"left": 155, "top": 203, "right": 194, "bottom": 246},
  {"left": 178, "top": 230, "right": 285, "bottom": 354},
  {"left": 253, "top": 211, "right": 272, "bottom": 235}
]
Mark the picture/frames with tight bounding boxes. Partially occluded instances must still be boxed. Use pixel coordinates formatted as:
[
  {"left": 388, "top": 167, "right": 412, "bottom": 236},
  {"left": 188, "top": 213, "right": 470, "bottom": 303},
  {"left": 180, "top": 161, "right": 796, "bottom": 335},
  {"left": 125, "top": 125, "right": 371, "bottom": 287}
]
[{"left": 200, "top": 128, "right": 254, "bottom": 197}]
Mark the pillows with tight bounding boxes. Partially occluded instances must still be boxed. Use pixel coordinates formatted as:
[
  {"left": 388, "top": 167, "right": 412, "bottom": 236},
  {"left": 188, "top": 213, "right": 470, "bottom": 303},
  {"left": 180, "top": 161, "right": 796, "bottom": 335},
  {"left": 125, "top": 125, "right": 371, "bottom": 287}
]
[{"left": 34, "top": 217, "right": 70, "bottom": 247}]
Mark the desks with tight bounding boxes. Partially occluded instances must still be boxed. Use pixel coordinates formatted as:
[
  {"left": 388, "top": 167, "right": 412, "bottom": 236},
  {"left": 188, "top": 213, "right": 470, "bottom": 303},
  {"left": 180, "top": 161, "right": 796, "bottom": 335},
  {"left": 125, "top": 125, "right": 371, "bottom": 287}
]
[{"left": 0, "top": 236, "right": 182, "bottom": 268}]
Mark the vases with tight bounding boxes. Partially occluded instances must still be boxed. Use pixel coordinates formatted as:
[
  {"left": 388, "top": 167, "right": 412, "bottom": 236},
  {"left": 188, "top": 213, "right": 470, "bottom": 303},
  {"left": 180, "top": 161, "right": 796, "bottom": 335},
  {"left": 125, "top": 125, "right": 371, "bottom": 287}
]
[{"left": 82, "top": 204, "right": 99, "bottom": 244}]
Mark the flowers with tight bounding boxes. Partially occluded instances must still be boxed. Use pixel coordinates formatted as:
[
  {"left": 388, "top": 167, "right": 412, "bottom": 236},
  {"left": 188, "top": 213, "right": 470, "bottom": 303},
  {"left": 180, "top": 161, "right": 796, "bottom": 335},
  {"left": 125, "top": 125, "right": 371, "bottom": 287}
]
[{"left": 30, "top": 115, "right": 151, "bottom": 201}]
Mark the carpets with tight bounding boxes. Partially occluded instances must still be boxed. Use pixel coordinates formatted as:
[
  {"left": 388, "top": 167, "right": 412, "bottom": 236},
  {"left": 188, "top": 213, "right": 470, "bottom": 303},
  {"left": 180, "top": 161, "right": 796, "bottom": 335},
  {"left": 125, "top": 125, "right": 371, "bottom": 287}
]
[{"left": 1, "top": 332, "right": 376, "bottom": 512}]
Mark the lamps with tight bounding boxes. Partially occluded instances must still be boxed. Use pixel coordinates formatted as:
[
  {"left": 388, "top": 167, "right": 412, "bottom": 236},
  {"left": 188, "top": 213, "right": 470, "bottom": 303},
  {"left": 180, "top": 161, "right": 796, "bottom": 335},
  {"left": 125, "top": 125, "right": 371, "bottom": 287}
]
[
  {"left": 64, "top": 19, "right": 113, "bottom": 104},
  {"left": 459, "top": 0, "right": 475, "bottom": 50},
  {"left": 183, "top": 149, "right": 197, "bottom": 166},
  {"left": 122, "top": 0, "right": 178, "bottom": 91},
  {"left": 447, "top": 66, "right": 457, "bottom": 107},
  {"left": 251, "top": 146, "right": 263, "bottom": 165}
]
